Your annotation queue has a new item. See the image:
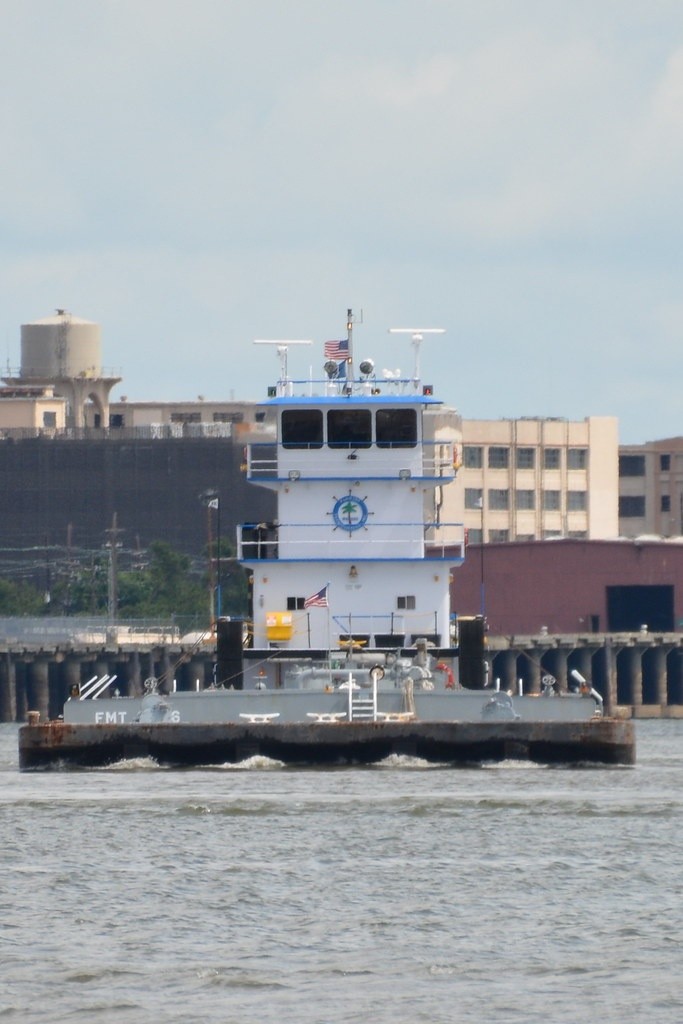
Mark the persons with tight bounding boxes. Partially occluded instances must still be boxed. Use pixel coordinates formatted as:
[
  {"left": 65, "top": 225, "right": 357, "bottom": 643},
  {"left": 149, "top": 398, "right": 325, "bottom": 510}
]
[{"left": 436, "top": 662, "right": 454, "bottom": 689}]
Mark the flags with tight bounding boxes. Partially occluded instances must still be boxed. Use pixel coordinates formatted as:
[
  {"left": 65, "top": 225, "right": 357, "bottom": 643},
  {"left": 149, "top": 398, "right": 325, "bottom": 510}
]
[
  {"left": 324, "top": 339, "right": 349, "bottom": 359},
  {"left": 208, "top": 499, "right": 218, "bottom": 510},
  {"left": 304, "top": 586, "right": 328, "bottom": 608},
  {"left": 474, "top": 497, "right": 483, "bottom": 509}
]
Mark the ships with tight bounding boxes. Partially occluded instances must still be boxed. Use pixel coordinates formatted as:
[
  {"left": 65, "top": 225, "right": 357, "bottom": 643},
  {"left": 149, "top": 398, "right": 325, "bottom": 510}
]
[{"left": 17, "top": 304, "right": 638, "bottom": 773}]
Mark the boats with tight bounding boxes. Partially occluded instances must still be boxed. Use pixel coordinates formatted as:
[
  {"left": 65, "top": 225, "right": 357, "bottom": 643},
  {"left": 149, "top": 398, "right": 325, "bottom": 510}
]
[{"left": 336, "top": 639, "right": 367, "bottom": 646}]
